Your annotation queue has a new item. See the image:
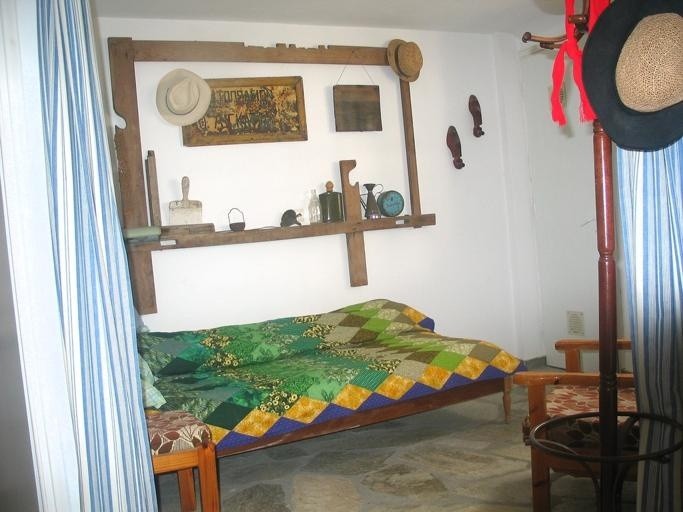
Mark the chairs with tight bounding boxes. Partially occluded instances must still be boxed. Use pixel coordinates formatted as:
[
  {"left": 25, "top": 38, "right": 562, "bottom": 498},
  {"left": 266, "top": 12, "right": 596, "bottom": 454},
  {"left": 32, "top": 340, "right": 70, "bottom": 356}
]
[
  {"left": 145, "top": 410, "right": 222, "bottom": 511},
  {"left": 513, "top": 339, "right": 639, "bottom": 511}
]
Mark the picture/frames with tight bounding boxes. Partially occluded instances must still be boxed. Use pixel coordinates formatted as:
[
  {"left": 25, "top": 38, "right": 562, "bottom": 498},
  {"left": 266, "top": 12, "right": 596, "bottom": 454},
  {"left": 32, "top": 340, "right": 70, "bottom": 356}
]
[{"left": 179, "top": 76, "right": 311, "bottom": 148}]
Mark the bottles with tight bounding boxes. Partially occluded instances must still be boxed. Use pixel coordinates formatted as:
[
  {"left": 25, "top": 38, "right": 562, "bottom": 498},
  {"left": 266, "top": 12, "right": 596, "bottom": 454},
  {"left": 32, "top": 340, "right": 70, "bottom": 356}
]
[{"left": 308, "top": 181, "right": 344, "bottom": 225}]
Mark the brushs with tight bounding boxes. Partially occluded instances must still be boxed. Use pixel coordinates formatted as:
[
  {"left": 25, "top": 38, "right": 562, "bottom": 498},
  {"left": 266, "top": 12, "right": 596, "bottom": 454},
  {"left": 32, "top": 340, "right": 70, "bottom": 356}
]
[{"left": 169, "top": 176, "right": 202, "bottom": 225}]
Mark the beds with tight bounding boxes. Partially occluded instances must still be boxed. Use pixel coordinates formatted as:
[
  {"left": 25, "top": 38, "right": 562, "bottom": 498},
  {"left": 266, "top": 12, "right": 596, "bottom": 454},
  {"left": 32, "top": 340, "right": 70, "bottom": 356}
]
[{"left": 130, "top": 298, "right": 521, "bottom": 465}]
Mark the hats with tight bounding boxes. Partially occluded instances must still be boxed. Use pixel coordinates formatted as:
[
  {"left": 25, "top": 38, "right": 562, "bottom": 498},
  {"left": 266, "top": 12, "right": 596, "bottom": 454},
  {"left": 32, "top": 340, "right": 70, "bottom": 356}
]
[
  {"left": 581, "top": 2, "right": 682, "bottom": 152},
  {"left": 386, "top": 38, "right": 424, "bottom": 83},
  {"left": 155, "top": 68, "right": 212, "bottom": 128}
]
